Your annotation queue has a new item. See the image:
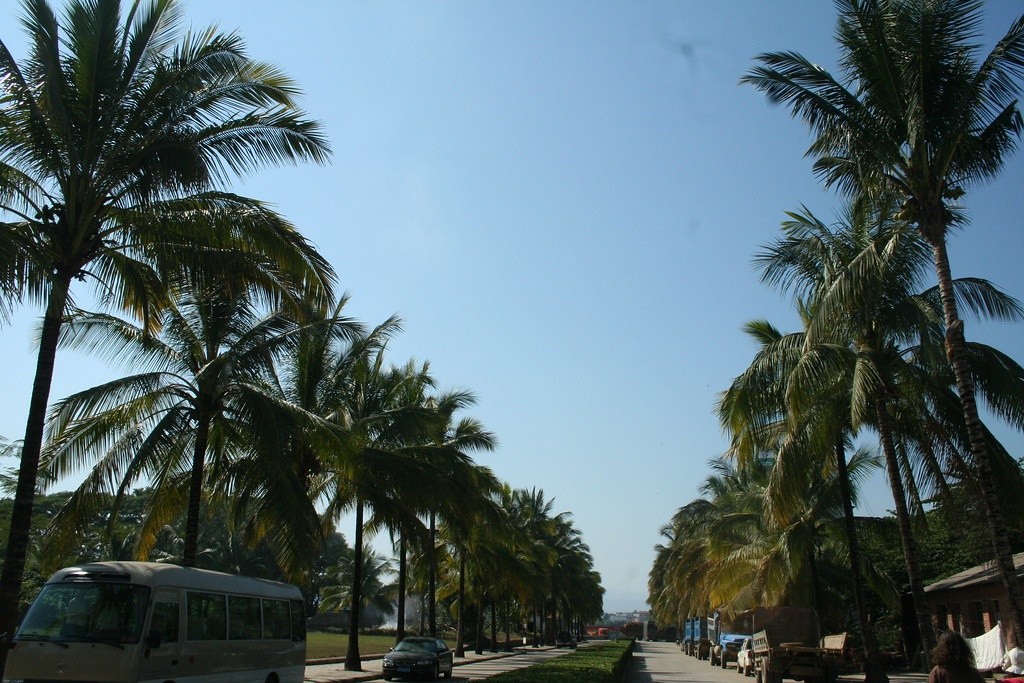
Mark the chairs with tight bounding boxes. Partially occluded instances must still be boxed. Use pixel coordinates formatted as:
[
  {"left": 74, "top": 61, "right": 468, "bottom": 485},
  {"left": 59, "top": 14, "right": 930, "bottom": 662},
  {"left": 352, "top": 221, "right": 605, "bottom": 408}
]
[{"left": 56, "top": 606, "right": 290, "bottom": 646}]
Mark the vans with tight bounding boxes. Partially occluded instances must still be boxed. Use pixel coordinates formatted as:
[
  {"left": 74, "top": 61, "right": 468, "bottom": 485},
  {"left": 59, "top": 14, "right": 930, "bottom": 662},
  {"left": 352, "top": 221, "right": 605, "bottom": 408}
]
[{"left": 2, "top": 560, "right": 306, "bottom": 683}]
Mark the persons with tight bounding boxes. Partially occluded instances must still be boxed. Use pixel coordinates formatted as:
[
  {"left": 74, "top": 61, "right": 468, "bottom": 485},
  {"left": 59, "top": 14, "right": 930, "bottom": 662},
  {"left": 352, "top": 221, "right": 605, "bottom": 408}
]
[{"left": 928, "top": 631, "right": 986, "bottom": 683}]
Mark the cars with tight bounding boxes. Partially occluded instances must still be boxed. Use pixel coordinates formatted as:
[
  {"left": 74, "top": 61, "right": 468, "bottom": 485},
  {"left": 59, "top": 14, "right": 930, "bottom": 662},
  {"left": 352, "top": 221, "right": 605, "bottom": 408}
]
[
  {"left": 556, "top": 630, "right": 576, "bottom": 649},
  {"left": 383, "top": 636, "right": 452, "bottom": 681}
]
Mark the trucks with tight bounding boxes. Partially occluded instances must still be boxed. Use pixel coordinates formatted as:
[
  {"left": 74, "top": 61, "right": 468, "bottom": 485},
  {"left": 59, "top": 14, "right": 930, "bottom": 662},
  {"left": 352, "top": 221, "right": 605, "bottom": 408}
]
[{"left": 682, "top": 604, "right": 852, "bottom": 683}]
